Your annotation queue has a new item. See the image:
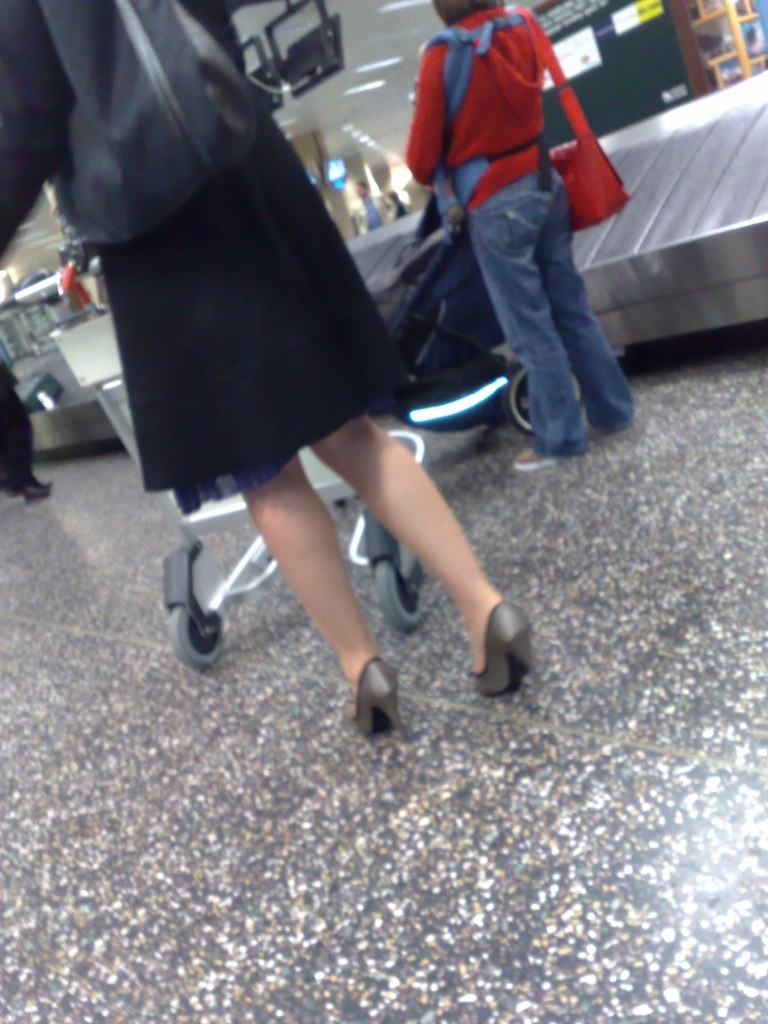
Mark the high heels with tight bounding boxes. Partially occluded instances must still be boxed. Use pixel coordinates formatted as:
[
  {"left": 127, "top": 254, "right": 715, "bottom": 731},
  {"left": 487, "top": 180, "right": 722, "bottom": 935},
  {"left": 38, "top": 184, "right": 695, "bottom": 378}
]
[
  {"left": 467, "top": 601, "right": 537, "bottom": 698},
  {"left": 346, "top": 657, "right": 401, "bottom": 739}
]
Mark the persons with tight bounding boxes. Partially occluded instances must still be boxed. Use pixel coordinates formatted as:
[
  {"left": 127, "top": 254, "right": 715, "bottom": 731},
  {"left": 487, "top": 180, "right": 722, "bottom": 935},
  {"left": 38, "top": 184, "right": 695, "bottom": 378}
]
[
  {"left": 405, "top": 0, "right": 634, "bottom": 468},
  {"left": 0, "top": 0, "right": 538, "bottom": 734},
  {"left": 351, "top": 180, "right": 407, "bottom": 234},
  {"left": 62, "top": 252, "right": 93, "bottom": 311},
  {"left": 0, "top": 356, "right": 52, "bottom": 499}
]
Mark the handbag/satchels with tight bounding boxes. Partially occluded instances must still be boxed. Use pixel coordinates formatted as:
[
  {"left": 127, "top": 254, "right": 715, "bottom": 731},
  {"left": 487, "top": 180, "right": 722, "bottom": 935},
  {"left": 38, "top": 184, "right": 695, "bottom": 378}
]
[
  {"left": 547, "top": 133, "right": 630, "bottom": 231},
  {"left": 38, "top": 0, "right": 258, "bottom": 245}
]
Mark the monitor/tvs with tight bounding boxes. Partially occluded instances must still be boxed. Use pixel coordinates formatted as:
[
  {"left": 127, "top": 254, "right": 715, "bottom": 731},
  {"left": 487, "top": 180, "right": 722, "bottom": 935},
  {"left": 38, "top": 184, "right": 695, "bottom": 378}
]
[
  {"left": 288, "top": 14, "right": 344, "bottom": 97},
  {"left": 250, "top": 59, "right": 283, "bottom": 111}
]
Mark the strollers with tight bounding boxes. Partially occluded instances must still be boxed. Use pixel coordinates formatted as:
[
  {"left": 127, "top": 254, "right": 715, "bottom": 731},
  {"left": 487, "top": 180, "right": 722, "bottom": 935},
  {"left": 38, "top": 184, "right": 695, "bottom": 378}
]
[
  {"left": 98, "top": 247, "right": 449, "bottom": 673},
  {"left": 350, "top": 188, "right": 544, "bottom": 437}
]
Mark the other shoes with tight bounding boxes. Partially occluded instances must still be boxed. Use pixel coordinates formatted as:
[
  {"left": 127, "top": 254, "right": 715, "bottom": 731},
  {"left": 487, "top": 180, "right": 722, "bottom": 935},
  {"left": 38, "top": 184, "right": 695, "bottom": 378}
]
[{"left": 22, "top": 484, "right": 52, "bottom": 502}]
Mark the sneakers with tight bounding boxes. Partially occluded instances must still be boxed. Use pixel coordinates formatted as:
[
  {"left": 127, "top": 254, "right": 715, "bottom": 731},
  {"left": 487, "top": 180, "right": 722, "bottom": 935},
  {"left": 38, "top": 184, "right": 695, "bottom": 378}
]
[{"left": 512, "top": 447, "right": 557, "bottom": 472}]
